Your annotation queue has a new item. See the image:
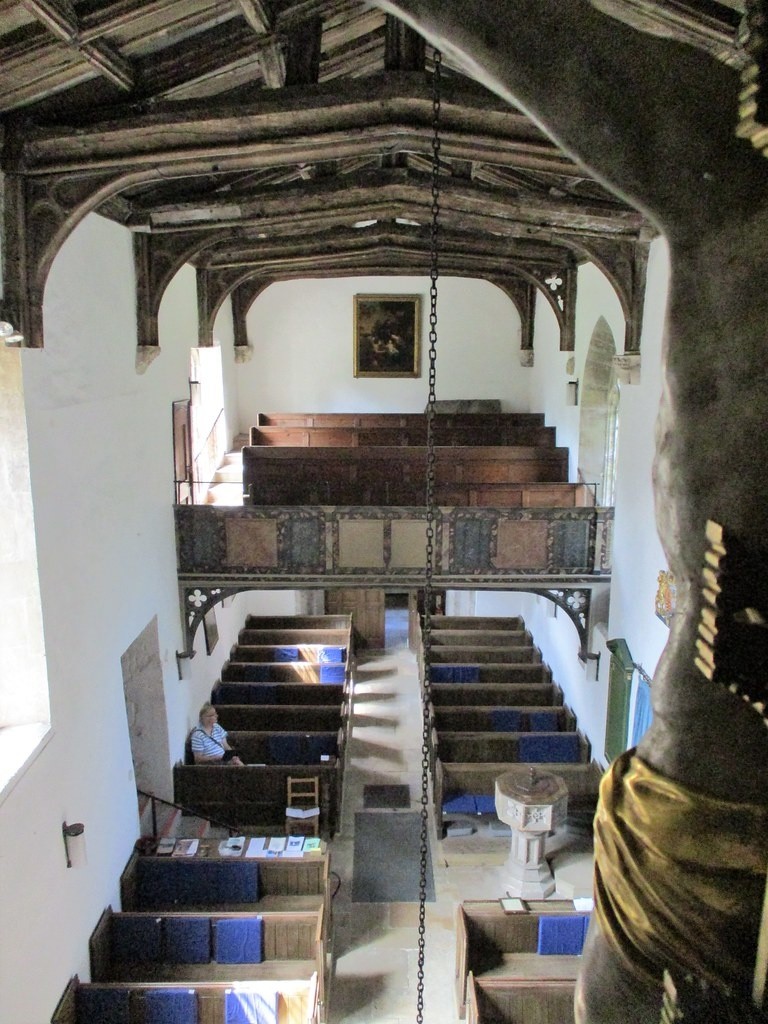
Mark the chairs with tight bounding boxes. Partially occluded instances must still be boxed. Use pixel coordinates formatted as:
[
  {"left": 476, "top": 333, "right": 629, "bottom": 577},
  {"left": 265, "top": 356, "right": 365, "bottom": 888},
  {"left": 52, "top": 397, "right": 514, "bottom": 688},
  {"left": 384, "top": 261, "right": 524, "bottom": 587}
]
[{"left": 285, "top": 775, "right": 319, "bottom": 838}]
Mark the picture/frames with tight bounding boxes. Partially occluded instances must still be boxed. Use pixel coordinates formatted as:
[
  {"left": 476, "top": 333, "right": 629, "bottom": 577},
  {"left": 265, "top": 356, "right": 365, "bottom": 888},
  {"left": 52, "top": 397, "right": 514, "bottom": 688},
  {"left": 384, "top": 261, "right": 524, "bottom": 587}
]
[
  {"left": 353, "top": 293, "right": 423, "bottom": 379},
  {"left": 203, "top": 607, "right": 220, "bottom": 656}
]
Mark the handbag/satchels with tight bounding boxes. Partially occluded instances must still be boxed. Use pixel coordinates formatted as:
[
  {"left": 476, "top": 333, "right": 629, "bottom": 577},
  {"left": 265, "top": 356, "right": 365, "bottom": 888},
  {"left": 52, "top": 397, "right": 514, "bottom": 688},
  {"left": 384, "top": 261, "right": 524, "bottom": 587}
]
[{"left": 222, "top": 750, "right": 238, "bottom": 761}]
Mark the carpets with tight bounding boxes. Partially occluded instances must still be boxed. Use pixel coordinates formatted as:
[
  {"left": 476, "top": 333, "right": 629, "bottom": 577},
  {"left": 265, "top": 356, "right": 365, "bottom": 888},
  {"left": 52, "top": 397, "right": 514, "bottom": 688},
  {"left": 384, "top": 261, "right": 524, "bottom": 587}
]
[
  {"left": 352, "top": 811, "right": 436, "bottom": 903},
  {"left": 363, "top": 784, "right": 410, "bottom": 809}
]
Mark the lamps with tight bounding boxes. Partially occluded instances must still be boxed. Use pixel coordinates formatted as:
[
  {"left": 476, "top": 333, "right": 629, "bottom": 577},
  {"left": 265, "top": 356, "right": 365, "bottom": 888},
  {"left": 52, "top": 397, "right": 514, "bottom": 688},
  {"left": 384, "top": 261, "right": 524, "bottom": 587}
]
[
  {"left": 565, "top": 379, "right": 579, "bottom": 406},
  {"left": 62, "top": 821, "right": 86, "bottom": 869}
]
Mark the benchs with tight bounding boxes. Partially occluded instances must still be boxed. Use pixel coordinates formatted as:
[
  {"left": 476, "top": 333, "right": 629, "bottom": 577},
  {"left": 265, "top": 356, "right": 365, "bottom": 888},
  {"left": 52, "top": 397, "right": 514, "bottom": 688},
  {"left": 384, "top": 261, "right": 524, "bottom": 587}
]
[
  {"left": 173, "top": 613, "right": 356, "bottom": 834},
  {"left": 456, "top": 898, "right": 591, "bottom": 1024},
  {"left": 52, "top": 853, "right": 332, "bottom": 1024},
  {"left": 417, "top": 612, "right": 606, "bottom": 841}
]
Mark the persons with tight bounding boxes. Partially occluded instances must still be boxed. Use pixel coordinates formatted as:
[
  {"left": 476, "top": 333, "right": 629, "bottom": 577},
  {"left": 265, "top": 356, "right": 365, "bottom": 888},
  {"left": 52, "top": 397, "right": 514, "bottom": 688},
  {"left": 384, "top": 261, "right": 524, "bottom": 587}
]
[
  {"left": 191, "top": 705, "right": 242, "bottom": 765},
  {"left": 572, "top": 213, "right": 768, "bottom": 1024}
]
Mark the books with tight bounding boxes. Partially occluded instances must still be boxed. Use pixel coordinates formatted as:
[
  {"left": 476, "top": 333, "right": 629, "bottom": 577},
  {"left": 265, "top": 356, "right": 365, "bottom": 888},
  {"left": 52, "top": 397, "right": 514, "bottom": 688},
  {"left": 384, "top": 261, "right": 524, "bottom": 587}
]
[
  {"left": 157, "top": 838, "right": 199, "bottom": 857},
  {"left": 218, "top": 837, "right": 245, "bottom": 856},
  {"left": 285, "top": 805, "right": 320, "bottom": 818},
  {"left": 499, "top": 897, "right": 527, "bottom": 914},
  {"left": 573, "top": 897, "right": 593, "bottom": 910},
  {"left": 246, "top": 836, "right": 320, "bottom": 858}
]
